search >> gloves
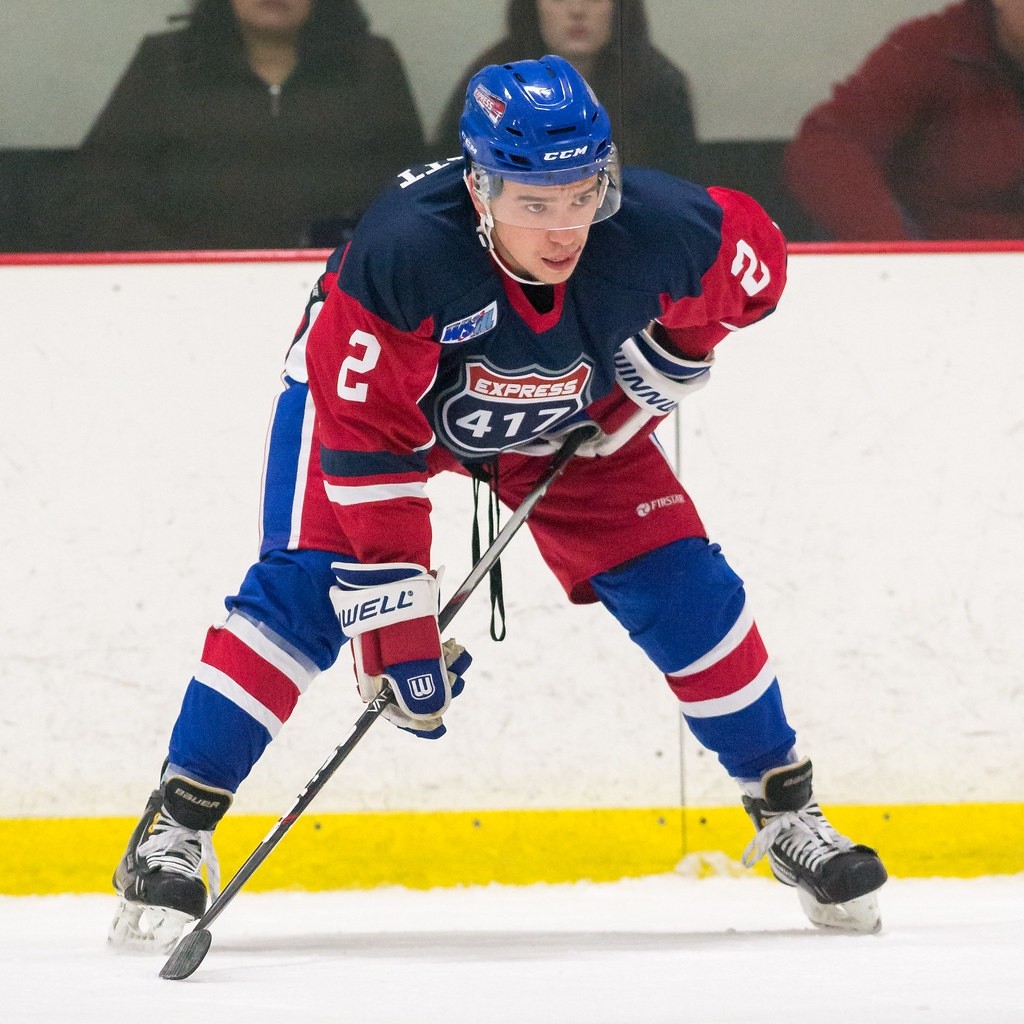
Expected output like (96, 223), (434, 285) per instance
(329, 560), (472, 740)
(540, 329), (715, 459)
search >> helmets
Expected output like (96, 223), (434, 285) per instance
(458, 54), (612, 205)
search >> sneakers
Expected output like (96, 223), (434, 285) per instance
(740, 755), (888, 934)
(107, 753), (233, 958)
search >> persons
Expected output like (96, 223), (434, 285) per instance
(82, 0), (424, 257)
(114, 57), (890, 962)
(431, 1), (702, 179)
(777, 1), (1024, 240)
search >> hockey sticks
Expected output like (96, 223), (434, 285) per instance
(160, 426), (595, 978)
